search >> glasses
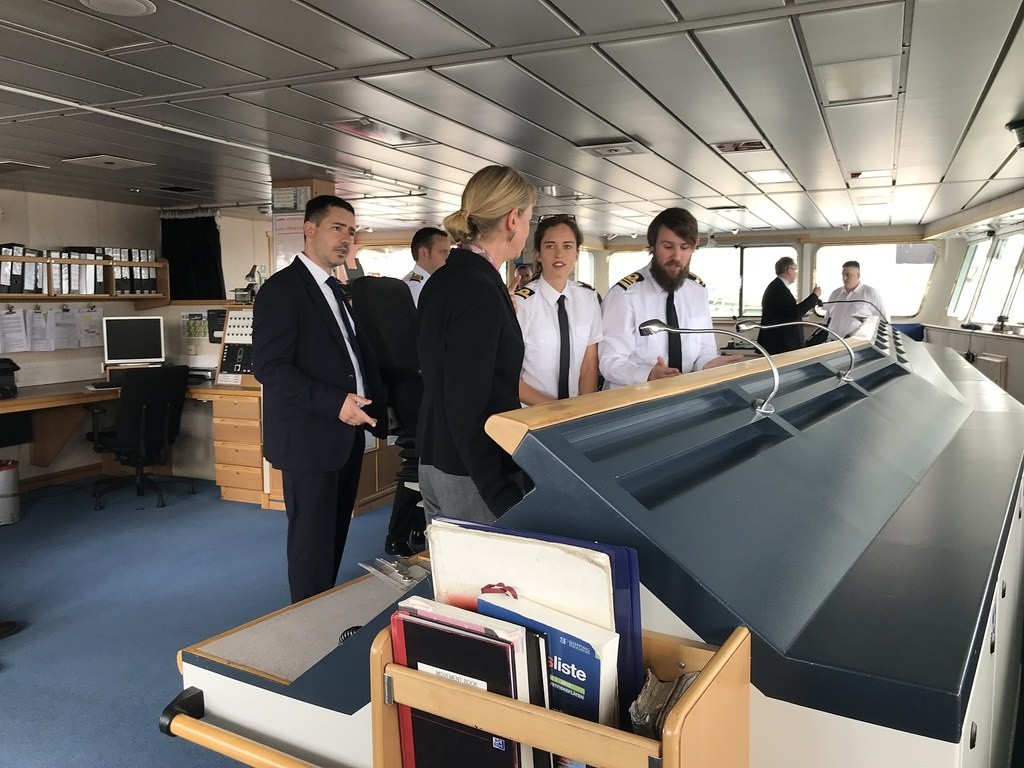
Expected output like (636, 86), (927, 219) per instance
(537, 213), (577, 225)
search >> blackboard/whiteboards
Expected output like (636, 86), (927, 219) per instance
(219, 342), (254, 374)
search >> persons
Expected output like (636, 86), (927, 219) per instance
(253, 195), (378, 604)
(414, 165), (537, 549)
(514, 265), (534, 288)
(385, 225), (451, 559)
(755, 257), (822, 355)
(514, 214), (604, 408)
(821, 260), (886, 341)
(597, 207), (744, 391)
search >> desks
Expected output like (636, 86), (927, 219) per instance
(719, 341), (763, 358)
(0, 378), (263, 505)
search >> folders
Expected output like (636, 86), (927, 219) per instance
(0, 242), (157, 294)
(430, 513), (644, 734)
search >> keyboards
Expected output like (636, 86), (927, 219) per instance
(91, 380), (121, 390)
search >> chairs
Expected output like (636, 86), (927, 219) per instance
(80, 365), (195, 511)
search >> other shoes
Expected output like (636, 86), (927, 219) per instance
(384, 535), (422, 557)
(0, 620), (23, 639)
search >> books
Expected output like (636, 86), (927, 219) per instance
(388, 591), (620, 768)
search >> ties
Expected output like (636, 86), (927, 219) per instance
(558, 295), (570, 400)
(666, 292), (682, 375)
(326, 276), (374, 411)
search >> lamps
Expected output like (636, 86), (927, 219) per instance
(245, 265), (261, 286)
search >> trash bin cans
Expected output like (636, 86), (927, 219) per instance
(0, 459), (21, 525)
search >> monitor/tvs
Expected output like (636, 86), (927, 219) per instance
(102, 316), (165, 367)
